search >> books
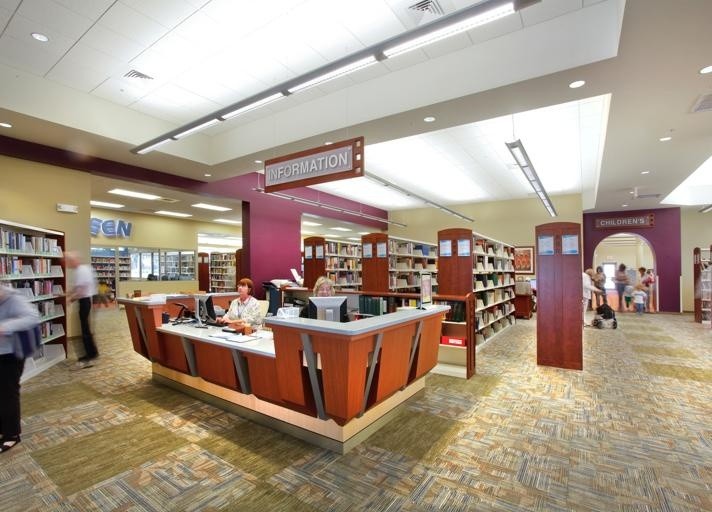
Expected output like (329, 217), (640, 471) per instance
(210, 254), (236, 292)
(358, 296), (466, 321)
(324, 242), (362, 290)
(0, 222), (131, 367)
(389, 240), (439, 307)
(473, 237), (516, 343)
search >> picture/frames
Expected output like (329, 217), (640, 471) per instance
(515, 246), (534, 274)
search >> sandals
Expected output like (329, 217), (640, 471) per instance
(1, 435), (20, 452)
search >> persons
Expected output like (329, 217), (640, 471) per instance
(0, 286), (41, 451)
(612, 263), (656, 314)
(216, 278), (261, 326)
(299, 276), (335, 318)
(583, 267), (603, 327)
(592, 267), (607, 308)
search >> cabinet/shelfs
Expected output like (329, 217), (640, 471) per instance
(91, 248), (243, 309)
(0, 219), (65, 385)
(535, 222), (584, 371)
(694, 245), (712, 323)
(281, 228), (515, 380)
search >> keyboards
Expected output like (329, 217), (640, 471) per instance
(208, 323), (225, 327)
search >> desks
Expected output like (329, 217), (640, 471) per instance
(515, 296), (533, 320)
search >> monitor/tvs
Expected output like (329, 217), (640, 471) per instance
(308, 296), (347, 322)
(194, 295), (217, 328)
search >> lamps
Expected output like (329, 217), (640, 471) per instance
(251, 173), (408, 228)
(129, 0), (544, 155)
(505, 139), (557, 218)
(365, 168), (475, 224)
